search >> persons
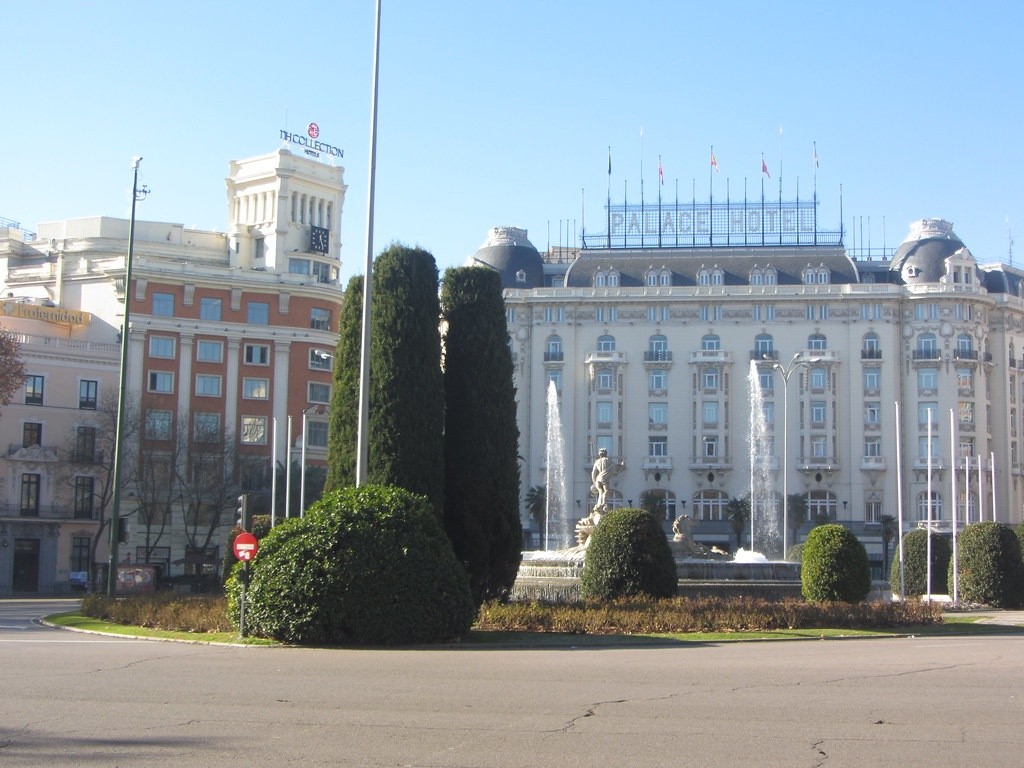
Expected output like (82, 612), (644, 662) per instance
(590, 447), (626, 514)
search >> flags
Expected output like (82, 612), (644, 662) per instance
(814, 148), (819, 167)
(762, 159), (771, 179)
(711, 152), (720, 172)
(658, 161), (664, 185)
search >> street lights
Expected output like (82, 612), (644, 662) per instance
(762, 351), (821, 561)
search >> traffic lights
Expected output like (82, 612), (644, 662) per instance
(236, 494), (253, 532)
